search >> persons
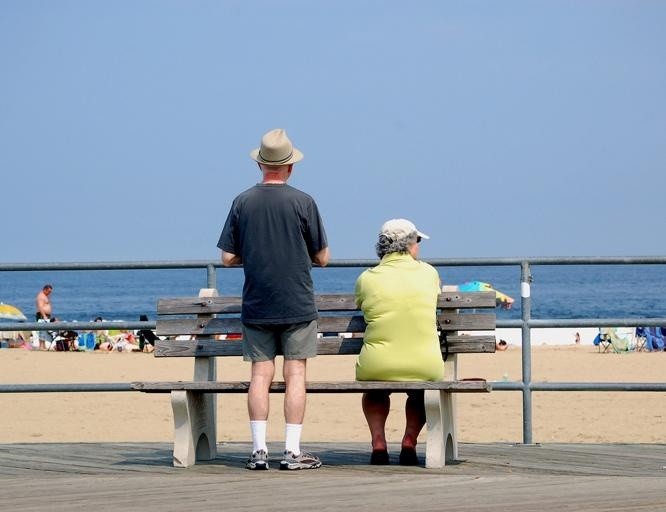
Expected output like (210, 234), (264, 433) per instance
(353, 218), (447, 466)
(214, 129), (330, 471)
(575, 332), (581, 344)
(34, 284), (54, 351)
(11, 316), (154, 353)
(495, 339), (509, 351)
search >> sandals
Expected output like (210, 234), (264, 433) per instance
(371, 450), (390, 464)
(398, 447), (419, 464)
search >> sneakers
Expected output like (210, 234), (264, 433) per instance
(279, 449), (323, 469)
(245, 449), (269, 470)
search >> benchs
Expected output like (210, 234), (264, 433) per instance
(130, 290), (497, 468)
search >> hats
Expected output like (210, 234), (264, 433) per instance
(378, 218), (431, 244)
(249, 128), (304, 166)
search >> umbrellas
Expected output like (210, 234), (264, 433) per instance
(0, 301), (28, 322)
(442, 280), (515, 314)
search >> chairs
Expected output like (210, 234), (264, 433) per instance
(48, 330), (78, 352)
(636, 326), (666, 352)
(599, 326), (635, 353)
